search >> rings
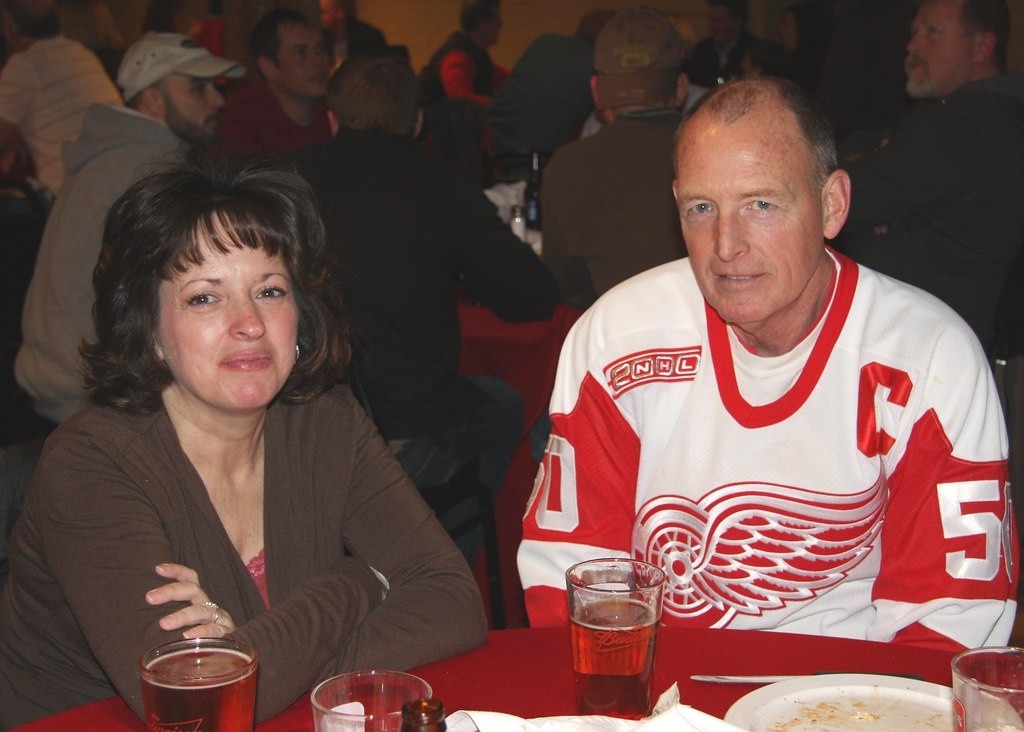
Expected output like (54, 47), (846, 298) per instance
(205, 602), (218, 622)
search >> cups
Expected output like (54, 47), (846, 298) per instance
(310, 669), (433, 732)
(141, 637), (258, 732)
(565, 557), (666, 721)
(951, 647), (1024, 732)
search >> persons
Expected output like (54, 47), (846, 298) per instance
(543, 8), (694, 306)
(516, 76), (1018, 652)
(0, 0), (1023, 569)
(281, 52), (556, 563)
(0, 165), (487, 732)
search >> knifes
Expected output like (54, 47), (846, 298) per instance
(690, 670), (925, 683)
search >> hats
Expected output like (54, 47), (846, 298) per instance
(116, 33), (244, 103)
(594, 5), (681, 109)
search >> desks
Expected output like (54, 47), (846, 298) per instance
(11, 623), (1024, 732)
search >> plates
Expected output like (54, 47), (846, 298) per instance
(723, 673), (952, 732)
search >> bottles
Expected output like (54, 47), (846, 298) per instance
(401, 698), (447, 732)
